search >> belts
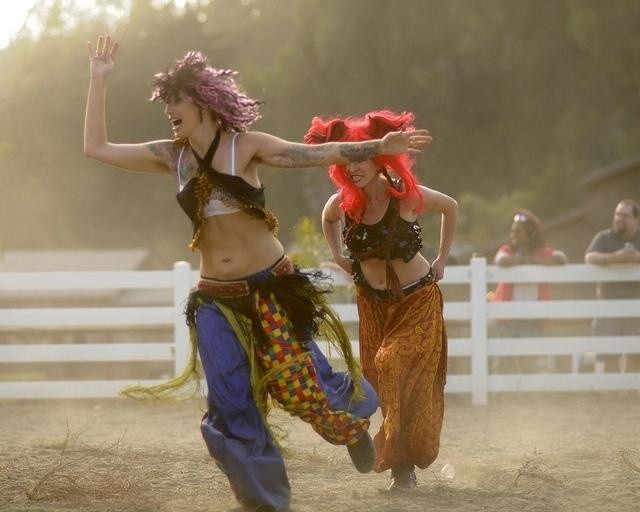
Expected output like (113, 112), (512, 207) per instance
(349, 267), (433, 299)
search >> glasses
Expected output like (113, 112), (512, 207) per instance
(512, 214), (530, 225)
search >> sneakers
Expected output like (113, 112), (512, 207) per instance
(347, 432), (377, 474)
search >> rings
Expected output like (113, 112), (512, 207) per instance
(95, 51), (102, 54)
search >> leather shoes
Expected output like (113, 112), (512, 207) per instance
(389, 465), (416, 492)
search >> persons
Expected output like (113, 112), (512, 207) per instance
(492, 208), (568, 374)
(583, 198), (640, 397)
(305, 110), (459, 495)
(81, 34), (433, 510)
